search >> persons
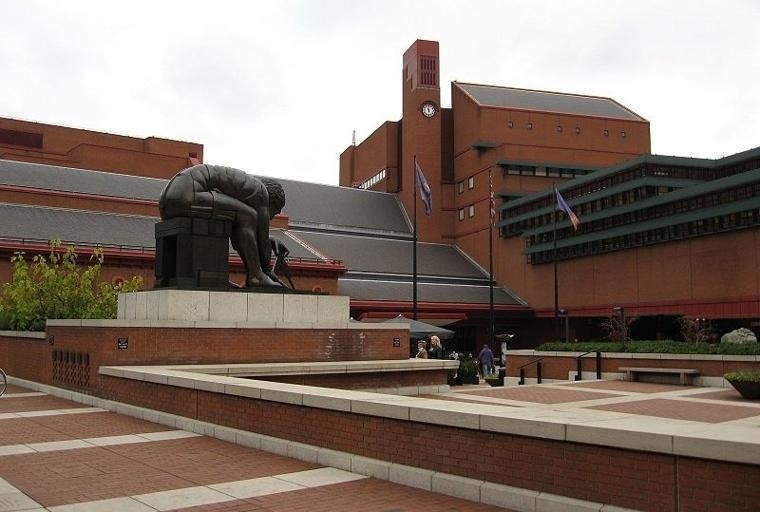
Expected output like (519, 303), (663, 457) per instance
(478, 343), (494, 378)
(456, 352), (466, 361)
(415, 340), (428, 359)
(157, 165), (291, 290)
(427, 335), (443, 359)
(450, 350), (460, 360)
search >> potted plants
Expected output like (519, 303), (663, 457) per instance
(724, 370), (760, 399)
(484, 372), (499, 387)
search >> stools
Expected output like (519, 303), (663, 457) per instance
(154, 216), (232, 290)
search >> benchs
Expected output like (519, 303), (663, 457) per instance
(618, 367), (700, 385)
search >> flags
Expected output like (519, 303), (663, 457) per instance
(413, 160), (434, 217)
(554, 186), (581, 232)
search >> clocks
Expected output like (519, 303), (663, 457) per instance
(422, 103), (435, 118)
